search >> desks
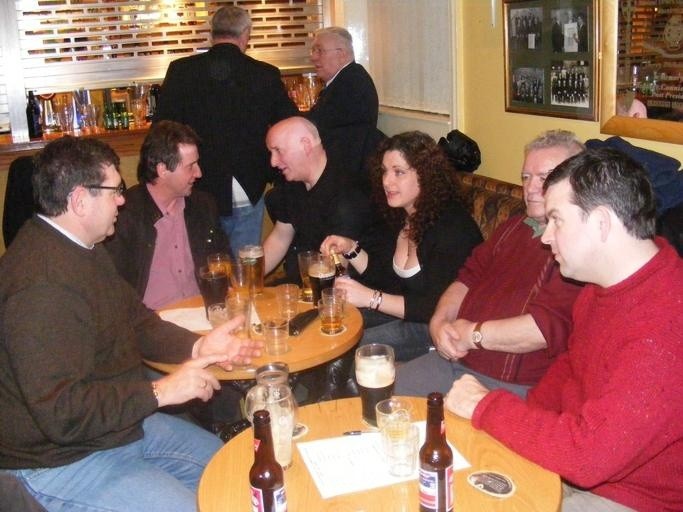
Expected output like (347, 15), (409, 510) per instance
(137, 284), (364, 405)
(196, 394), (564, 512)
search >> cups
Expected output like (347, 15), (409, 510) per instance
(352, 345), (398, 425)
(207, 252), (232, 278)
(207, 304), (230, 328)
(253, 359), (289, 398)
(297, 251), (315, 301)
(379, 424), (418, 476)
(131, 99), (145, 128)
(236, 245), (267, 295)
(199, 264), (225, 307)
(225, 290), (252, 328)
(322, 288), (345, 312)
(315, 300), (344, 336)
(245, 381), (293, 474)
(309, 256), (336, 304)
(261, 317), (292, 357)
(275, 283), (300, 318)
(373, 398), (413, 435)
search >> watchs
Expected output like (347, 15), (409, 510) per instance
(473, 320), (485, 352)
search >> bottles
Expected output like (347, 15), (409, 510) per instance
(630, 65), (639, 92)
(249, 408), (285, 512)
(25, 90), (42, 139)
(102, 100), (129, 130)
(641, 75), (653, 95)
(415, 391), (454, 512)
(58, 92), (75, 134)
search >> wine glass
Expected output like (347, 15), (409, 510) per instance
(90, 104), (99, 130)
(78, 101), (89, 129)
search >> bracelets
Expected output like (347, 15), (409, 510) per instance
(342, 241), (362, 261)
(150, 379), (161, 405)
(375, 289), (382, 312)
(367, 288), (379, 311)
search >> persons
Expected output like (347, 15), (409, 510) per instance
(259, 116), (393, 296)
(304, 131), (485, 401)
(616, 95), (649, 121)
(104, 121), (258, 440)
(1, 136), (264, 512)
(152, 7), (301, 282)
(367, 127), (590, 412)
(512, 12), (590, 108)
(442, 146), (683, 512)
(270, 27), (379, 190)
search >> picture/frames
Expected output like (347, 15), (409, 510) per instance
(502, 1), (596, 122)
(598, 0), (682, 145)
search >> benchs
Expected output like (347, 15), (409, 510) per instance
(394, 167), (525, 371)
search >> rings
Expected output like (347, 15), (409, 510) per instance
(202, 379), (208, 389)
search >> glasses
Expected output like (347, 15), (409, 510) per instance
(68, 184), (124, 197)
(311, 48), (342, 56)
